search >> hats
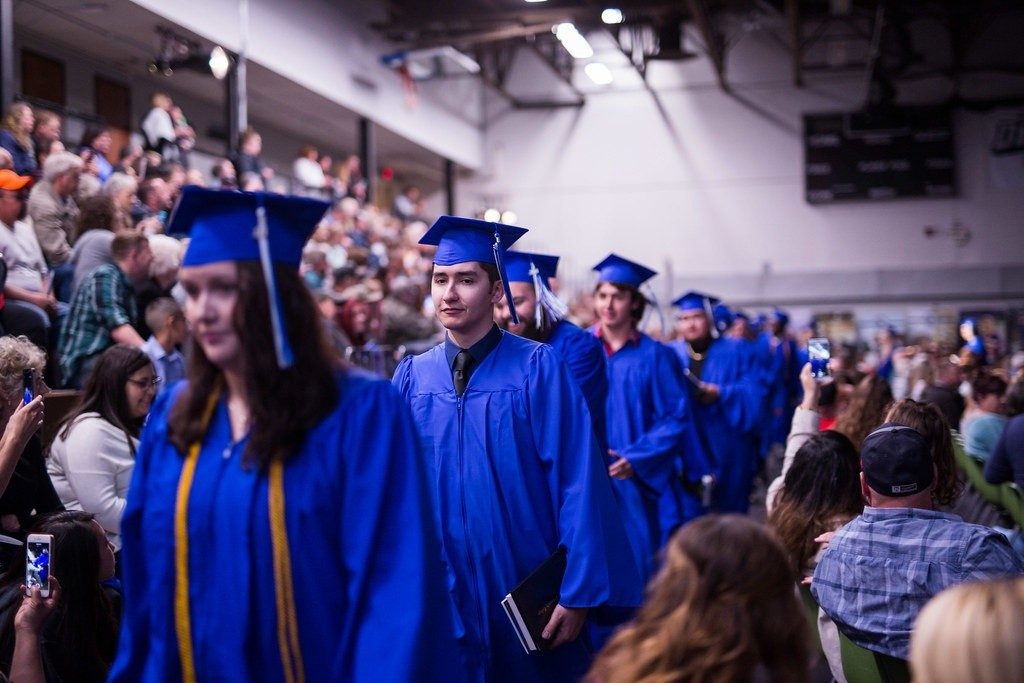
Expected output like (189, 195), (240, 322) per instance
(592, 254), (666, 338)
(503, 249), (568, 328)
(672, 292), (788, 339)
(0, 168), (33, 190)
(860, 422), (934, 498)
(164, 183), (333, 368)
(417, 214), (529, 324)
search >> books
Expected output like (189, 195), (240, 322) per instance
(501, 550), (567, 654)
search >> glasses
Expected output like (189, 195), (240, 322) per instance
(128, 374), (162, 388)
(2, 191), (30, 202)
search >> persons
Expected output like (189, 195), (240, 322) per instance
(0, 298), (188, 683)
(107, 183), (458, 683)
(391, 216), (645, 683)
(594, 253), (694, 654)
(34, 547), (48, 586)
(729, 308), (820, 484)
(495, 251), (609, 466)
(670, 292), (763, 515)
(582, 331), (1024, 683)
(0, 94), (597, 389)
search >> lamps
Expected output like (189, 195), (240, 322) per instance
(207, 45), (236, 82)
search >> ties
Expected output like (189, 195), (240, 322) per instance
(453, 353), (473, 398)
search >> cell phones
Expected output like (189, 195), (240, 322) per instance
(808, 337), (832, 383)
(23, 368), (37, 406)
(26, 533), (55, 598)
